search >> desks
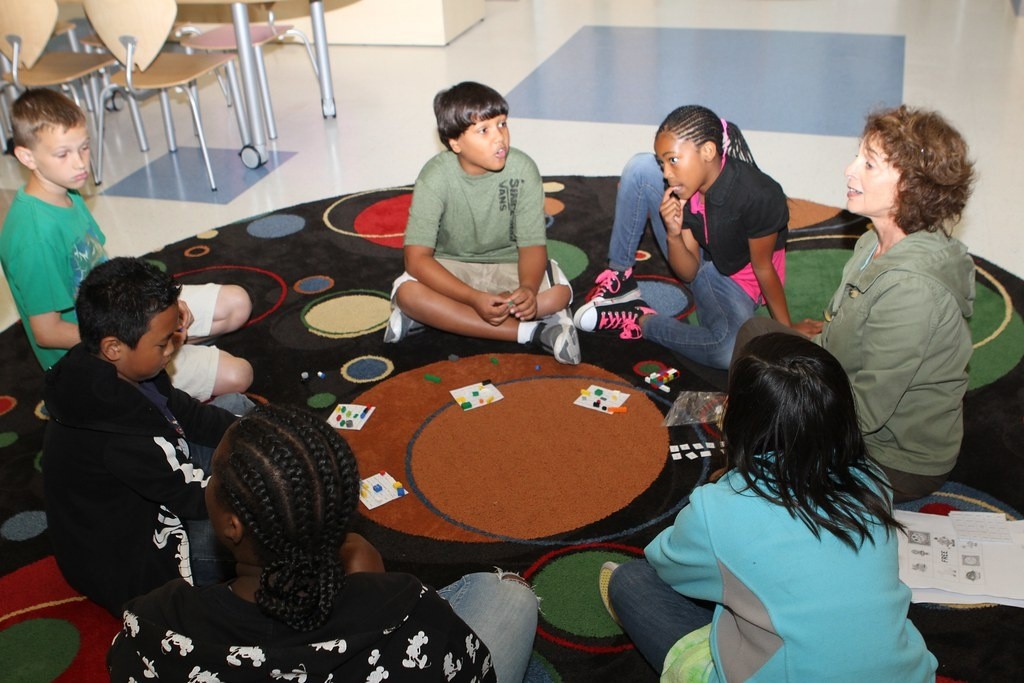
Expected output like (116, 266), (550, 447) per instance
(63, 0), (339, 168)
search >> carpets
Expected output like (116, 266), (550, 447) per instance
(0, 175), (1024, 683)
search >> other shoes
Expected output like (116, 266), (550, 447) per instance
(599, 562), (622, 627)
(574, 299), (657, 340)
(590, 267), (641, 302)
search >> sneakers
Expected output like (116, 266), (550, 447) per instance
(531, 308), (580, 367)
(384, 307), (426, 344)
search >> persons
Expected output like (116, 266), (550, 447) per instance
(597, 332), (940, 682)
(728, 104), (977, 503)
(35, 255), (260, 612)
(389, 82), (585, 365)
(1, 87), (254, 401)
(570, 104), (818, 373)
(101, 401), (545, 683)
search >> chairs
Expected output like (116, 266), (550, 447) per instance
(0, 0), (319, 191)
